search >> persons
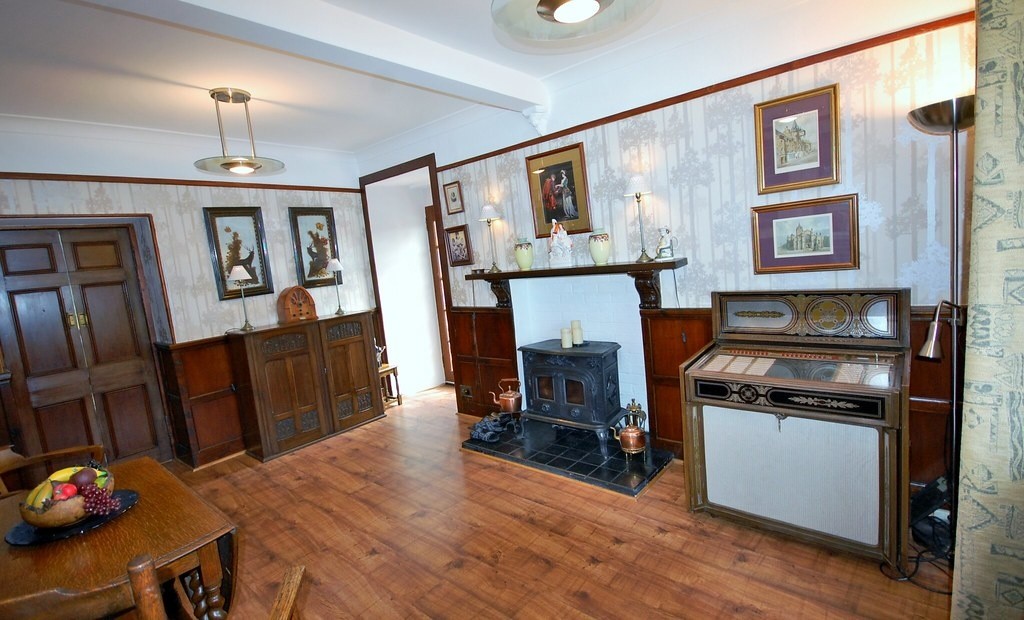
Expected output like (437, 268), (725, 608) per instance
(374, 337), (385, 367)
(546, 218), (573, 260)
(656, 224), (673, 257)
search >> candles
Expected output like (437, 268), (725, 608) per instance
(561, 319), (582, 349)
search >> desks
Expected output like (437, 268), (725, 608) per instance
(0, 457), (239, 620)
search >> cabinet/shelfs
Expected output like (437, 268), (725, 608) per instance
(225, 308), (388, 463)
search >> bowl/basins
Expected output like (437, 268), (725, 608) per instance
(20, 468), (115, 527)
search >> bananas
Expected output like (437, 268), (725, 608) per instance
(26, 467), (108, 509)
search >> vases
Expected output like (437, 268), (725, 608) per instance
(514, 237), (533, 271)
(589, 228), (610, 265)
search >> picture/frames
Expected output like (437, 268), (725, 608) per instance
(750, 193), (860, 275)
(525, 141), (594, 239)
(444, 224), (475, 267)
(443, 181), (465, 216)
(288, 207), (343, 289)
(202, 206), (274, 301)
(754, 82), (840, 195)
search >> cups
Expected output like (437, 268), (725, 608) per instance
(561, 328), (572, 348)
(571, 321), (583, 345)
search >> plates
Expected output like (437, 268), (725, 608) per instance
(4, 490), (140, 545)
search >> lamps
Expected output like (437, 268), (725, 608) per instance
(478, 206), (502, 273)
(906, 95), (975, 548)
(228, 265), (256, 331)
(491, 0), (660, 55)
(326, 259), (346, 315)
(192, 87), (286, 177)
(623, 175), (654, 263)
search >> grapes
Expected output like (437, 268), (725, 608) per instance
(79, 483), (121, 514)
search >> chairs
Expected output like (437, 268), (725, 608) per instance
(379, 363), (402, 412)
(0, 443), (106, 500)
(0, 553), (168, 619)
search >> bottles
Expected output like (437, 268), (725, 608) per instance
(589, 226), (609, 266)
(514, 237), (533, 271)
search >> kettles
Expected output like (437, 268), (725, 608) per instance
(610, 411), (647, 454)
(489, 378), (522, 412)
